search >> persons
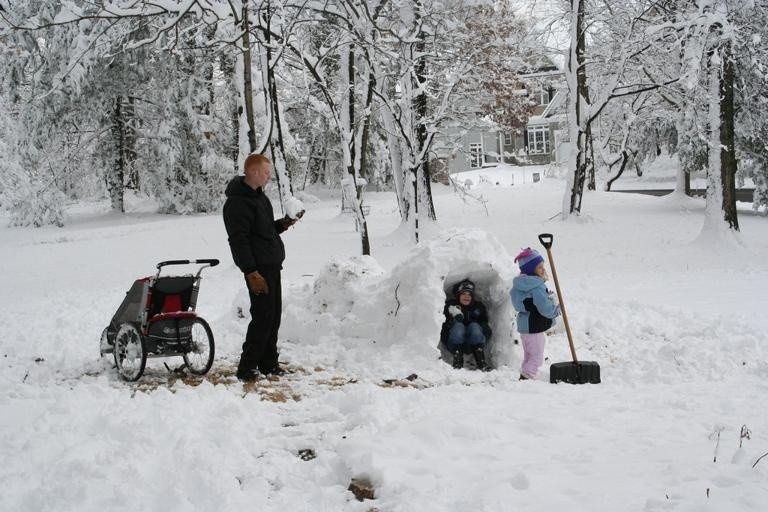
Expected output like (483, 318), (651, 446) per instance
(222, 154), (306, 382)
(508, 248), (560, 383)
(441, 280), (493, 372)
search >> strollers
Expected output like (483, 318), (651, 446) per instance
(100, 258), (219, 382)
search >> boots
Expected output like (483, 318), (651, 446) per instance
(452, 344), (463, 368)
(470, 343), (491, 371)
(237, 354), (286, 382)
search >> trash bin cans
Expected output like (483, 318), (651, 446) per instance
(533, 173), (540, 182)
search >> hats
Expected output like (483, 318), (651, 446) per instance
(455, 280), (474, 295)
(514, 247), (544, 275)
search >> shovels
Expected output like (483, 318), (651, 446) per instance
(539, 233), (600, 384)
